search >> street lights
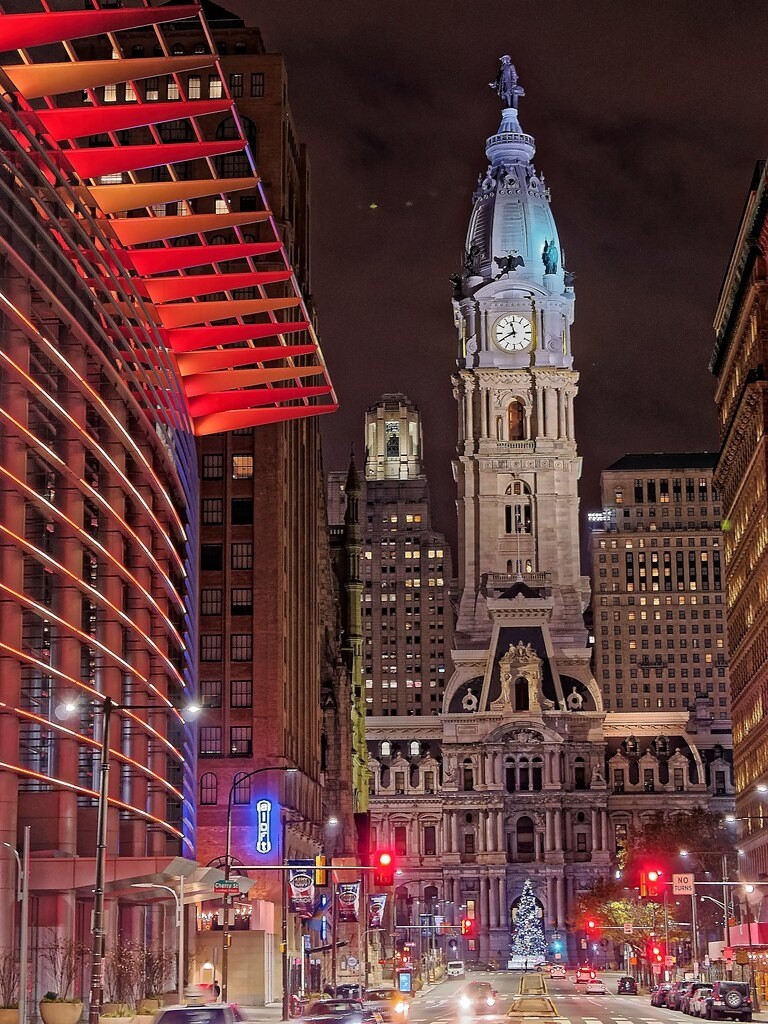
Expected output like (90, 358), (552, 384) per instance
(393, 877), (431, 988)
(280, 814), (338, 1021)
(130, 874), (185, 1006)
(222, 766), (298, 1004)
(357, 925), (386, 999)
(700, 885), (735, 919)
(677, 849), (744, 981)
(64, 697), (204, 1024)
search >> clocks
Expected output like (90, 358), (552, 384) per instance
(490, 311), (533, 354)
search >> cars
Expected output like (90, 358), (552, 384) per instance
(464, 960), (496, 972)
(697, 994), (714, 1020)
(650, 982), (674, 1008)
(534, 961), (558, 972)
(361, 986), (409, 1024)
(336, 984), (366, 1000)
(585, 979), (606, 996)
(463, 981), (499, 1015)
(688, 988), (714, 1017)
(293, 998), (385, 1024)
(550, 965), (566, 979)
(576, 967), (594, 984)
(156, 1004), (244, 1024)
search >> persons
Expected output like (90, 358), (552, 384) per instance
(211, 981), (220, 1000)
(488, 54), (524, 108)
(323, 985), (334, 998)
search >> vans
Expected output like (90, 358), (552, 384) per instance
(447, 961), (466, 980)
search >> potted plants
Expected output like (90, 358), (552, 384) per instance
(411, 978), (424, 992)
(0, 926), (179, 1024)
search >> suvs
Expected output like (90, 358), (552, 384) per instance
(617, 976), (638, 995)
(707, 981), (753, 1023)
(665, 980), (699, 1011)
(679, 982), (714, 1015)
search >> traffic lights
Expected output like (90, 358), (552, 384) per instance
(464, 919), (473, 936)
(647, 864), (660, 898)
(586, 918), (596, 934)
(649, 943), (665, 962)
(374, 849), (396, 887)
